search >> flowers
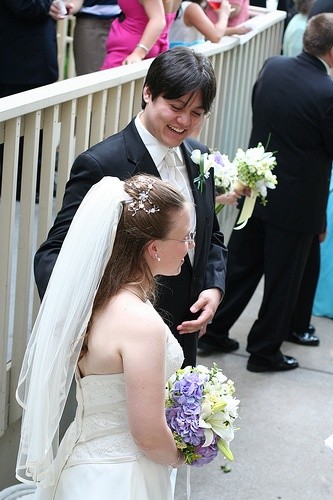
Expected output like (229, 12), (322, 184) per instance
(191, 149), (214, 193)
(209, 134), (278, 231)
(165, 361), (240, 474)
